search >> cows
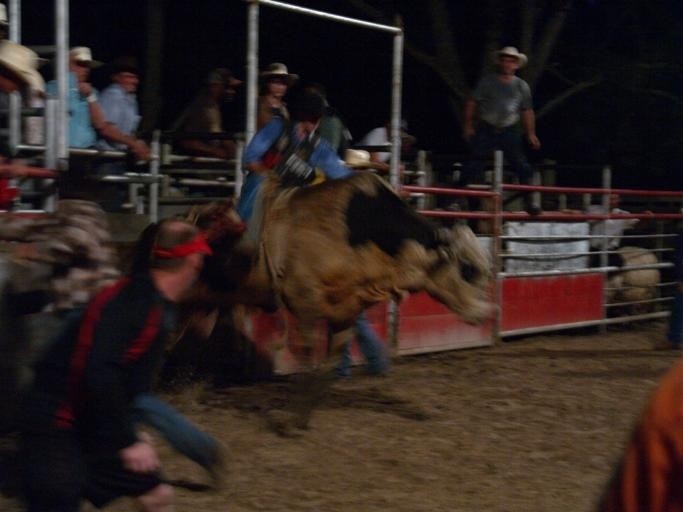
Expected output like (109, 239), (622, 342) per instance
(171, 168), (499, 441)
(577, 245), (660, 334)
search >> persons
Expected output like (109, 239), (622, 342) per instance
(337, 305), (384, 383)
(350, 115), (416, 183)
(594, 360), (682, 512)
(665, 208), (683, 349)
(13, 215), (228, 511)
(2, 38), (151, 214)
(584, 190), (653, 279)
(458, 45), (541, 209)
(172, 60), (355, 252)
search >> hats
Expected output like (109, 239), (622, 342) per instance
(0, 39), (45, 92)
(263, 63), (298, 81)
(71, 46), (105, 68)
(490, 47), (528, 68)
